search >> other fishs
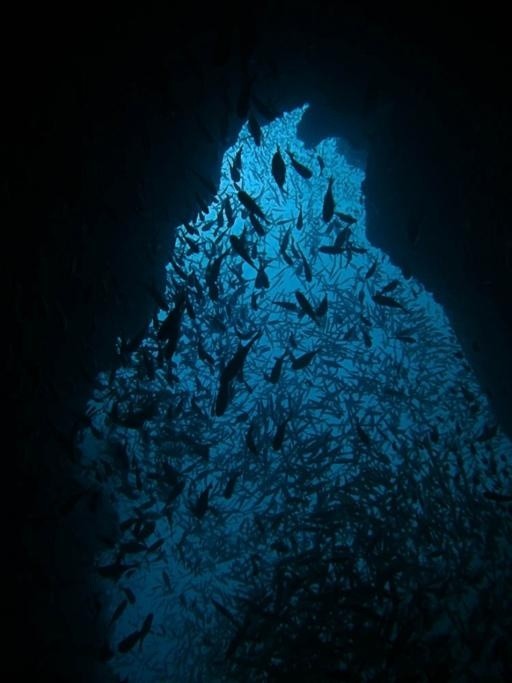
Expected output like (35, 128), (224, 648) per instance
(72, 106), (512, 683)
